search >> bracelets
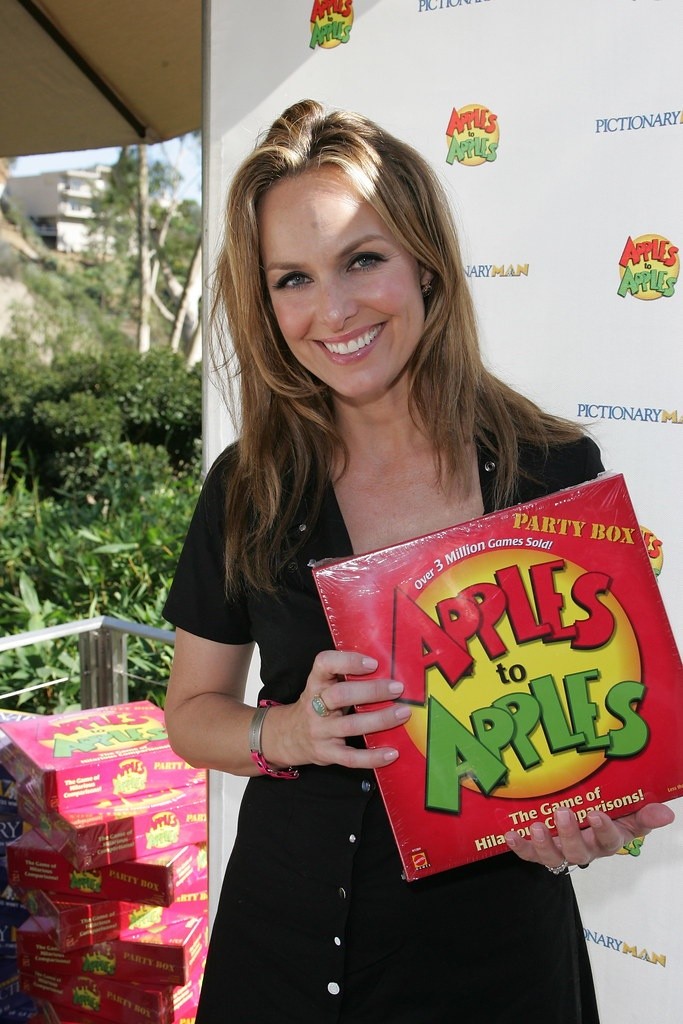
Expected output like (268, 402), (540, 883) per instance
(248, 700), (301, 780)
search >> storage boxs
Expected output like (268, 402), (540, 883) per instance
(16, 911), (209, 987)
(307, 470), (683, 882)
(9, 872), (208, 954)
(36, 995), (202, 1024)
(6, 829), (208, 908)
(17, 783), (210, 872)
(0, 700), (206, 813)
(18, 951), (208, 1024)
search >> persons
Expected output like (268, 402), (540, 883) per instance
(162, 100), (675, 1024)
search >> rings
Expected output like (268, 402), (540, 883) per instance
(313, 691), (337, 718)
(545, 859), (568, 876)
